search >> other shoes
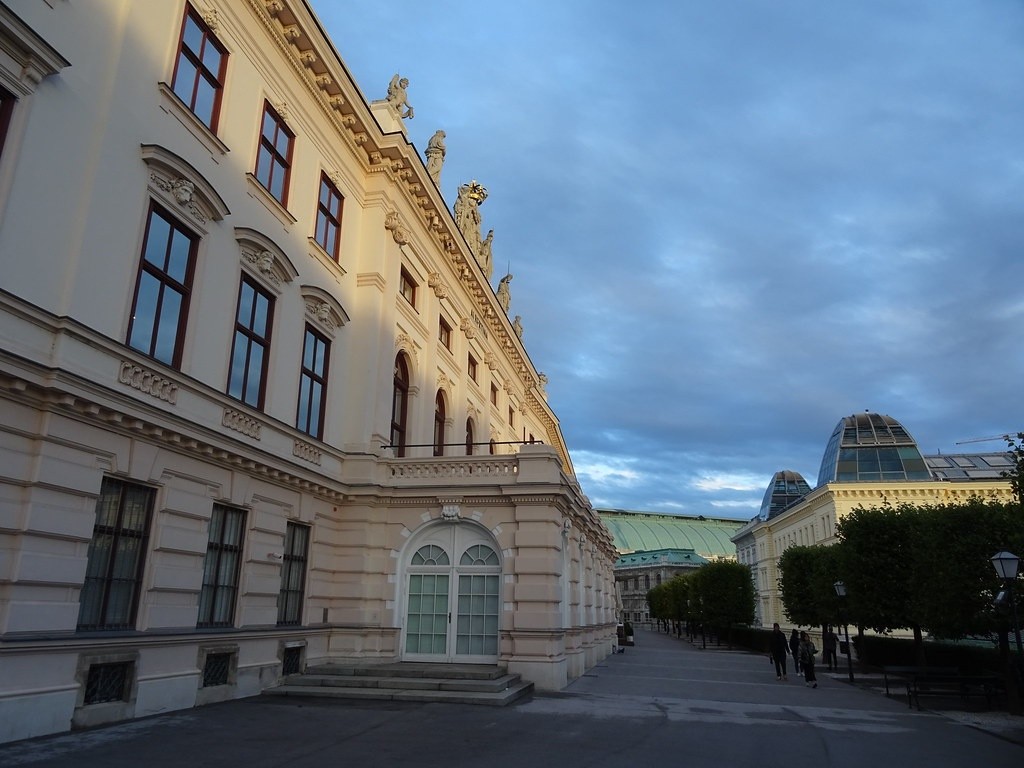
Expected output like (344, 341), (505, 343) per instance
(806, 682), (809, 687)
(777, 676), (781, 679)
(784, 674), (787, 679)
(812, 683), (817, 688)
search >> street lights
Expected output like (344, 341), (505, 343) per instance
(833, 579), (855, 681)
(989, 542), (1024, 665)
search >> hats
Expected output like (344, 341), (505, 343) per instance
(792, 629), (800, 634)
(829, 628), (833, 631)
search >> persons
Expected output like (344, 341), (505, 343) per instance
(424, 129), (447, 188)
(386, 74), (411, 117)
(767, 623), (790, 680)
(498, 274), (513, 311)
(826, 627), (840, 671)
(513, 316), (523, 337)
(797, 634), (818, 688)
(789, 629), (805, 674)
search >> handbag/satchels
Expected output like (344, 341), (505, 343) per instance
(770, 652), (773, 664)
